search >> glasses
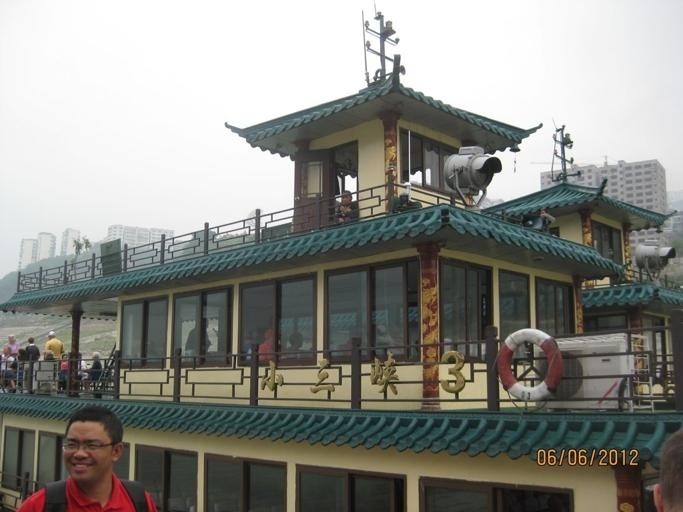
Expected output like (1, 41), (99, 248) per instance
(62, 438), (113, 451)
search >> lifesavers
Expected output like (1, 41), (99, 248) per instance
(496, 328), (563, 402)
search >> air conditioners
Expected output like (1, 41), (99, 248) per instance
(534, 331), (634, 412)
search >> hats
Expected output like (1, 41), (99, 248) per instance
(47, 331), (56, 337)
(265, 329), (281, 338)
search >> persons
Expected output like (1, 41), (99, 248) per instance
(652, 427), (682, 512)
(245, 327), (277, 361)
(0, 329), (102, 399)
(14, 404), (159, 511)
(335, 190), (359, 226)
(283, 330), (308, 360)
(184, 318), (213, 353)
